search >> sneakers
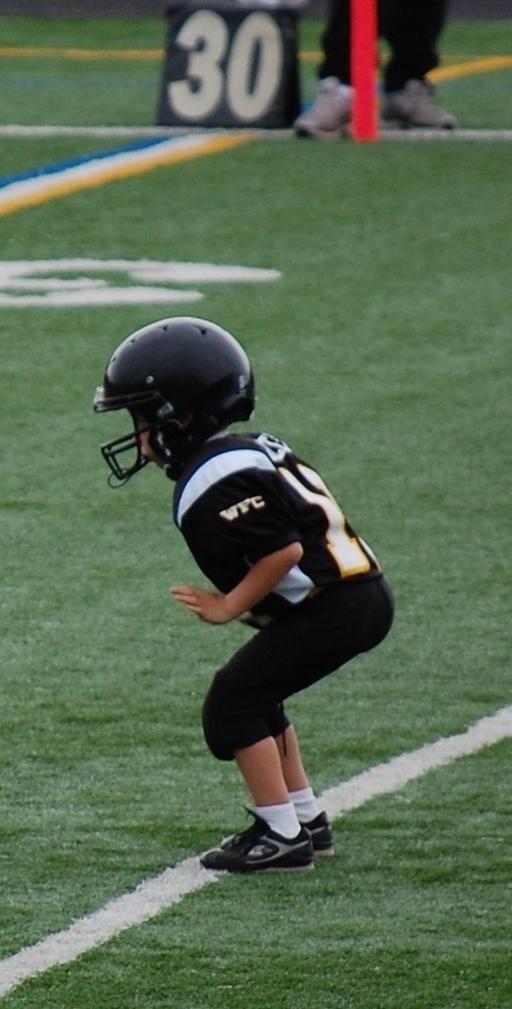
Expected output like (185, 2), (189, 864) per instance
(292, 74), (355, 142)
(196, 810), (336, 875)
(381, 80), (460, 133)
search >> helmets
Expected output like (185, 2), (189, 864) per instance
(90, 313), (256, 485)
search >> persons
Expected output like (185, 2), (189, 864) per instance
(294, 1), (459, 141)
(92, 315), (396, 873)
